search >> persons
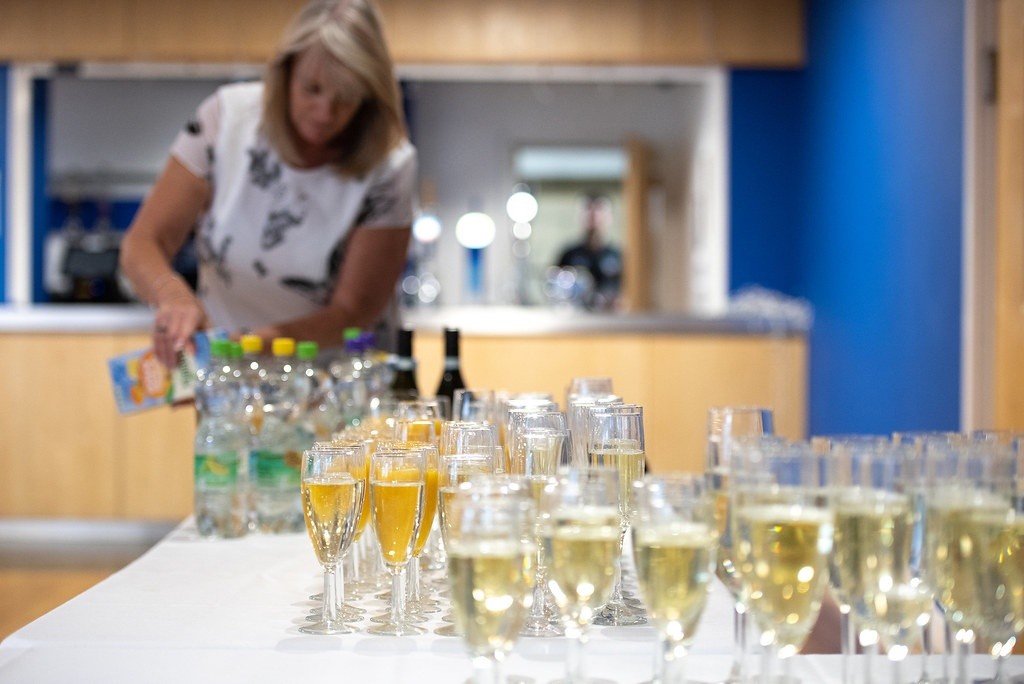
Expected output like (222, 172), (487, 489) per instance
(556, 194), (622, 311)
(121, 0), (422, 372)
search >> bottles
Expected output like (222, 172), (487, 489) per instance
(192, 325), (472, 538)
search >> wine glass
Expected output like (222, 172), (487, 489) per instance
(298, 375), (1024, 684)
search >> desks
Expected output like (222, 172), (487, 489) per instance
(0, 508), (1024, 684)
